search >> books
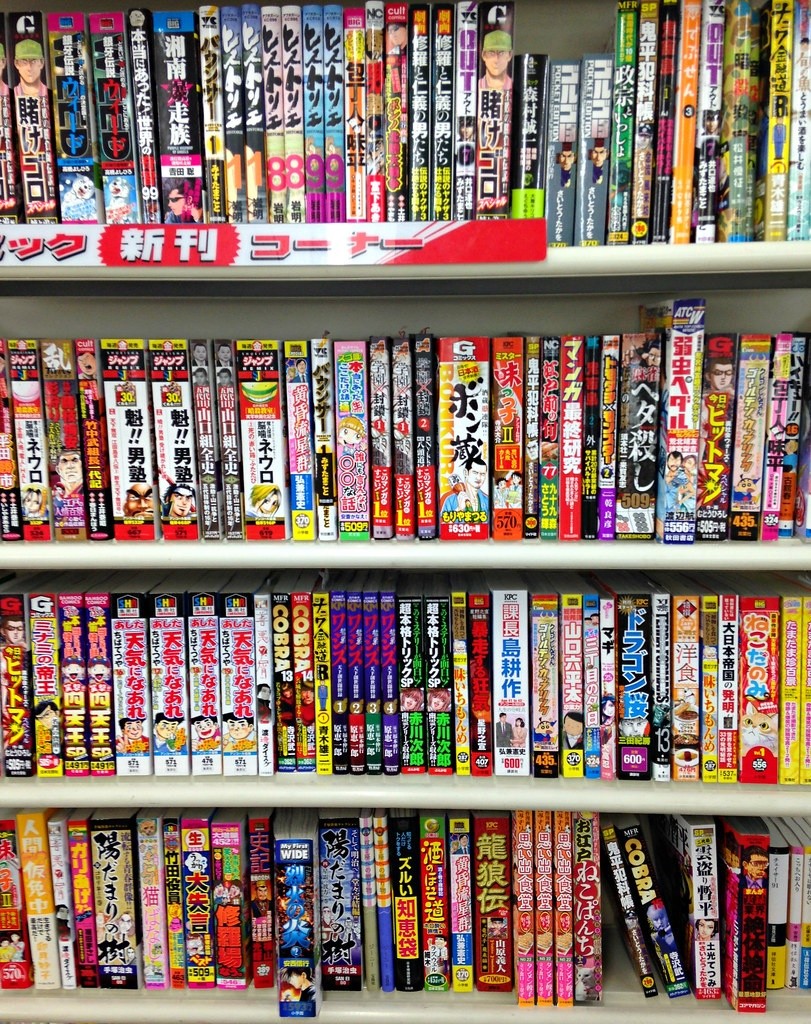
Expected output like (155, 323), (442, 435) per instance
(0, 571), (811, 789)
(0, 298), (811, 543)
(0, 808), (811, 1018)
(0, 0), (811, 249)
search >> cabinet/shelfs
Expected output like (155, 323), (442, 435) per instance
(0, 241), (811, 1024)
